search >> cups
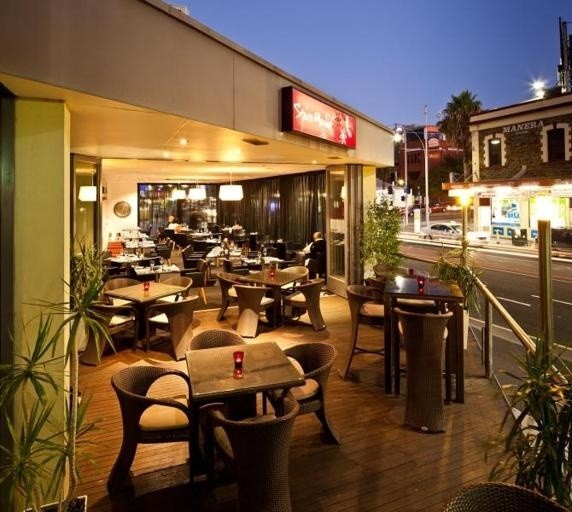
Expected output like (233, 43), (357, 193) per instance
(233, 351), (243, 368)
(418, 288), (424, 296)
(144, 281), (149, 291)
(234, 369), (243, 379)
(416, 276), (424, 288)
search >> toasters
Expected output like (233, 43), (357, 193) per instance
(150, 259), (172, 271)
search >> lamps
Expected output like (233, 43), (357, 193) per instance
(171, 161), (187, 201)
(187, 159), (208, 203)
(219, 154), (246, 202)
(79, 161), (98, 204)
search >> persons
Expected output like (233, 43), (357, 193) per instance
(304, 231), (324, 267)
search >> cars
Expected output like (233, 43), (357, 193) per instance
(138, 195), (217, 221)
(421, 222), (492, 250)
(387, 201), (462, 217)
(269, 193), (282, 213)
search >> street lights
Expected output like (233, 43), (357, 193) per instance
(393, 126), (409, 225)
(394, 105), (432, 228)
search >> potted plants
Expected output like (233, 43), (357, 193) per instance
(355, 191), (408, 280)
(39, 238), (115, 367)
(0, 254), (114, 512)
(433, 244), (478, 350)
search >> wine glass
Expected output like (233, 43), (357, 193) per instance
(258, 248), (267, 262)
(120, 248), (141, 259)
(221, 242), (225, 251)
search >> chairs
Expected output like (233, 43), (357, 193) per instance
(341, 264), (465, 433)
(80, 225), (339, 512)
